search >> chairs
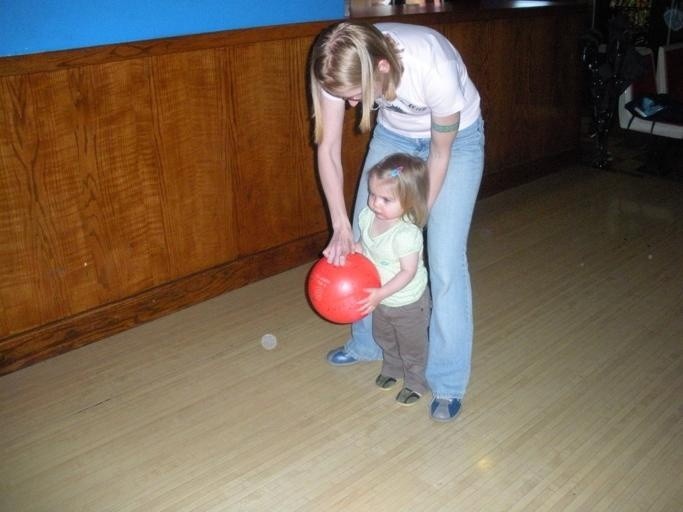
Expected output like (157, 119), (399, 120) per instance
(656, 42), (683, 103)
(616, 47), (683, 177)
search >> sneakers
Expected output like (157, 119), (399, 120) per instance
(375, 373), (398, 391)
(395, 386), (420, 405)
(326, 346), (358, 365)
(429, 397), (464, 422)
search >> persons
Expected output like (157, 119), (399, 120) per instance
(306, 18), (485, 422)
(345, 151), (432, 405)
